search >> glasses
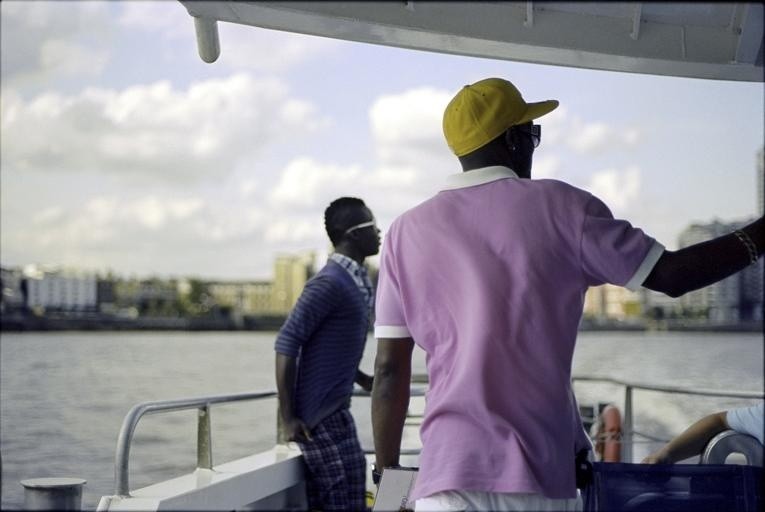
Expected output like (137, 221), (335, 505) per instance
(516, 123), (543, 148)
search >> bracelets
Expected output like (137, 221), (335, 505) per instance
(731, 229), (757, 266)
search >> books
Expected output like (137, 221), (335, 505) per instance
(373, 465), (419, 512)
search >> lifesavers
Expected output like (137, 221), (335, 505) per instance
(595, 406), (623, 461)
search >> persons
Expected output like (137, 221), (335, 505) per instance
(275, 199), (380, 512)
(636, 403), (765, 486)
(370, 79), (765, 512)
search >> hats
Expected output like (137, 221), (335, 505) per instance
(441, 78), (559, 157)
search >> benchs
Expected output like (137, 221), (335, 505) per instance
(590, 462), (765, 512)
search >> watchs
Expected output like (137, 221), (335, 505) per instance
(371, 462), (382, 485)
